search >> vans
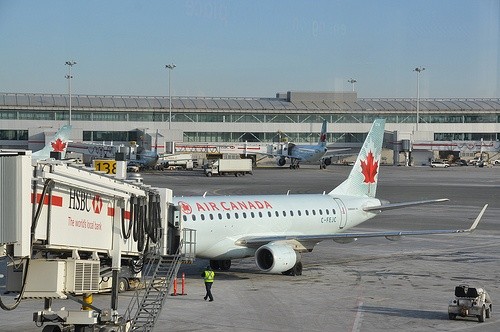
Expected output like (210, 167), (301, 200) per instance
(455, 159), (467, 165)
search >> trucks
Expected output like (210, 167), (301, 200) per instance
(204, 158), (253, 177)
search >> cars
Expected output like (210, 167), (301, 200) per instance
(446, 285), (493, 322)
(431, 161), (450, 168)
(127, 161), (139, 173)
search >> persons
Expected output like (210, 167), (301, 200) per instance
(201, 266), (216, 301)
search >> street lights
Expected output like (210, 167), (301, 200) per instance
(63, 60), (78, 126)
(163, 64), (176, 130)
(348, 79), (357, 93)
(410, 67), (426, 131)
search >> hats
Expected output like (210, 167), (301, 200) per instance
(207, 266), (211, 271)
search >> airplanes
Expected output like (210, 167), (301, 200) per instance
(130, 129), (198, 172)
(172, 118), (489, 277)
(243, 118), (352, 170)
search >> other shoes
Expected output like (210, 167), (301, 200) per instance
(204, 297), (206, 300)
(209, 299), (213, 301)
